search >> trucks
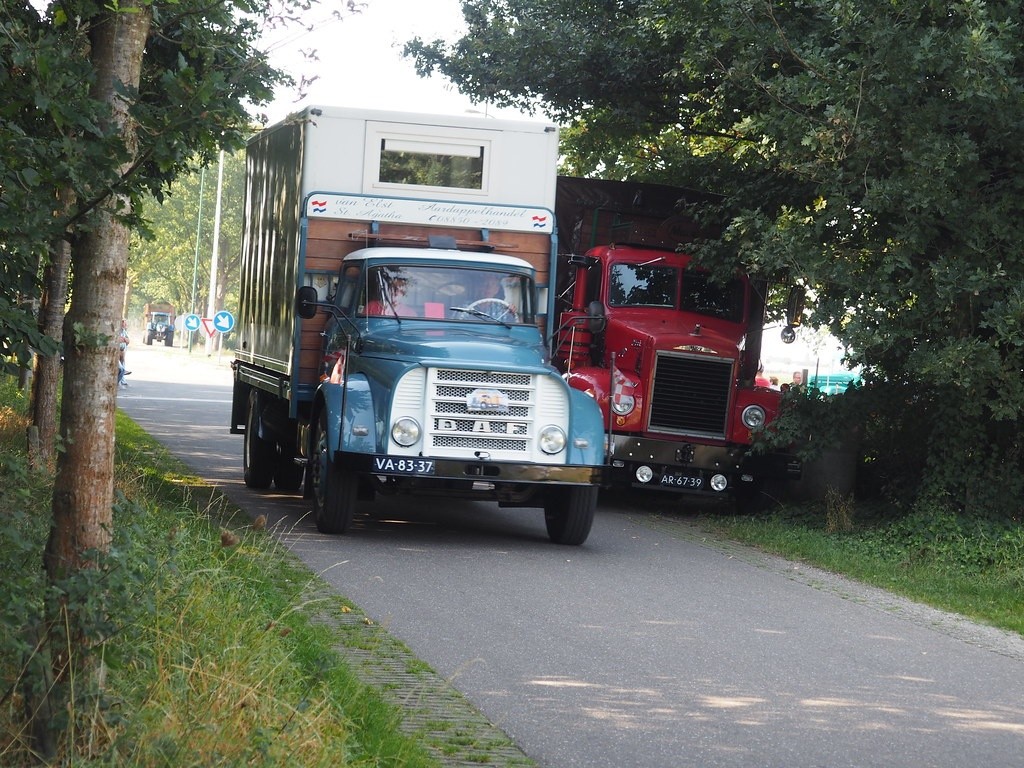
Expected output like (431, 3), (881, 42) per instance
(227, 104), (606, 545)
(142, 303), (175, 346)
(545, 174), (808, 514)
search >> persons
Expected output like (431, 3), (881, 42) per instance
(118, 318), (132, 386)
(365, 275), (418, 317)
(755, 364), (803, 393)
(453, 276), (519, 323)
